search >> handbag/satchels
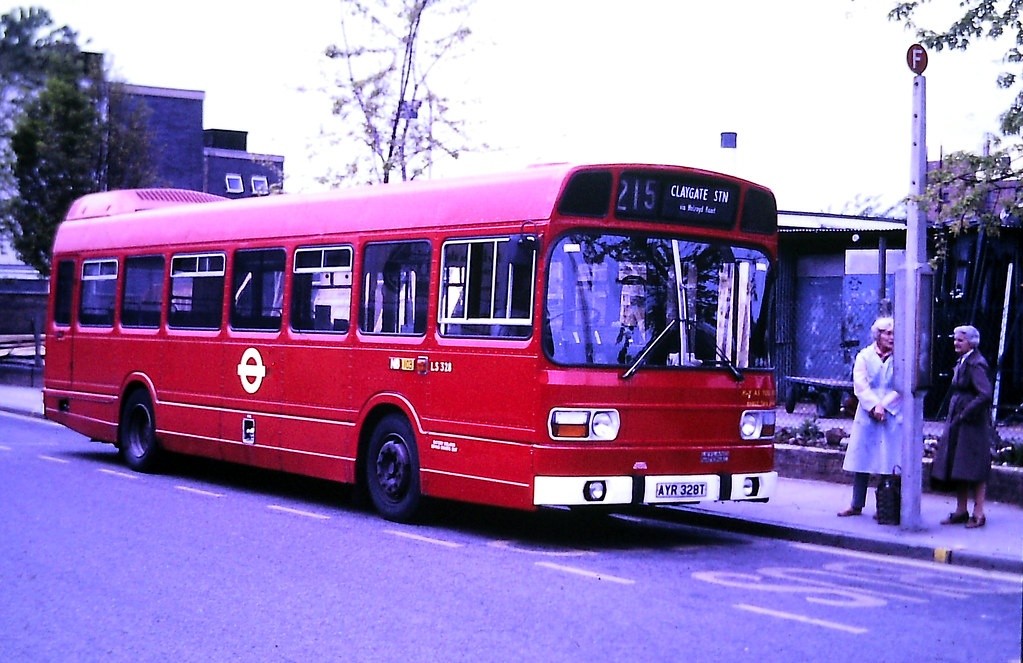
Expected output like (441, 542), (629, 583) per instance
(875, 466), (901, 526)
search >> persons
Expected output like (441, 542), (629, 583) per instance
(837, 317), (903, 519)
(931, 325), (994, 528)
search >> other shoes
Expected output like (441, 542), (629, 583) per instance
(965, 513), (986, 527)
(837, 506), (862, 516)
(940, 511), (969, 525)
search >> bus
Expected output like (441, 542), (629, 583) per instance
(41, 163), (780, 523)
